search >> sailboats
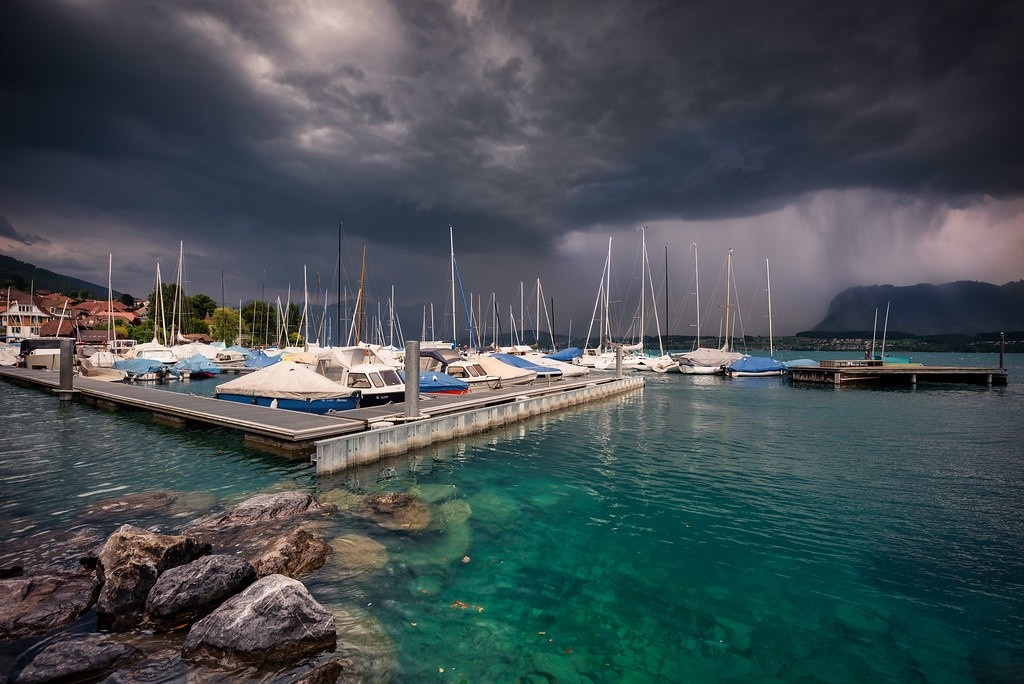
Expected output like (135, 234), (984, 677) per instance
(0, 222), (923, 412)
